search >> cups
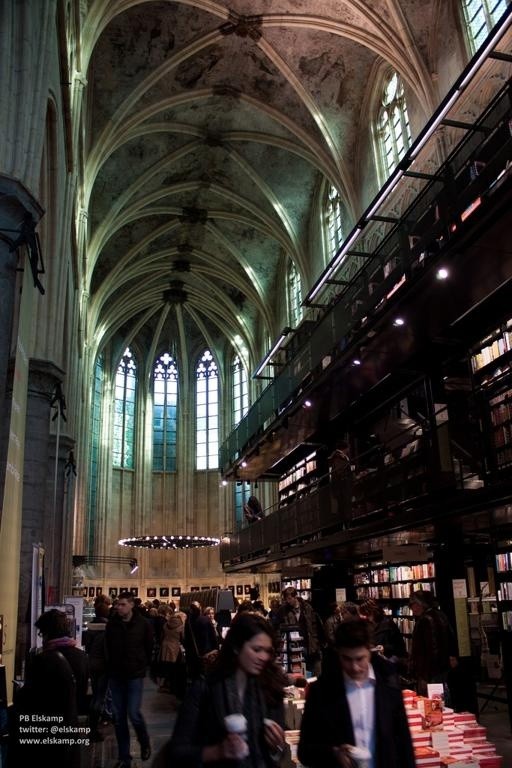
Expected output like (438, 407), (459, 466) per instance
(348, 747), (373, 768)
(225, 712), (251, 759)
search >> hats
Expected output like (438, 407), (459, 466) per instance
(32, 608), (68, 627)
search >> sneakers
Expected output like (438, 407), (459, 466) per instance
(115, 758), (132, 768)
(140, 742), (151, 761)
(86, 728), (105, 743)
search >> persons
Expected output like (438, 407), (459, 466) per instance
(328, 441), (353, 529)
(244, 496), (265, 524)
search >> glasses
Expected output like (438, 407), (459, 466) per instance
(36, 630), (45, 638)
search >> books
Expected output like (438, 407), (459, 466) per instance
(470, 331), (512, 466)
(278, 461), (317, 509)
